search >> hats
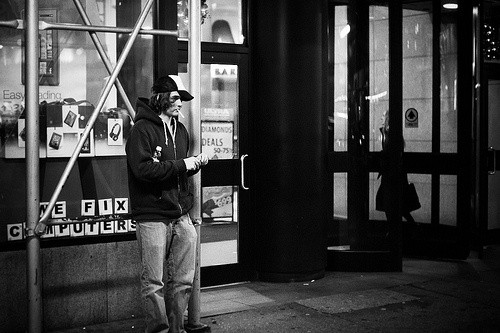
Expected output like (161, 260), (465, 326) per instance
(152, 74), (194, 102)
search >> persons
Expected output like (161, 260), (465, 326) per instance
(125, 74), (208, 333)
(376, 111), (421, 252)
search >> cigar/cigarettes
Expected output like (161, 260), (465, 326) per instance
(177, 109), (185, 118)
